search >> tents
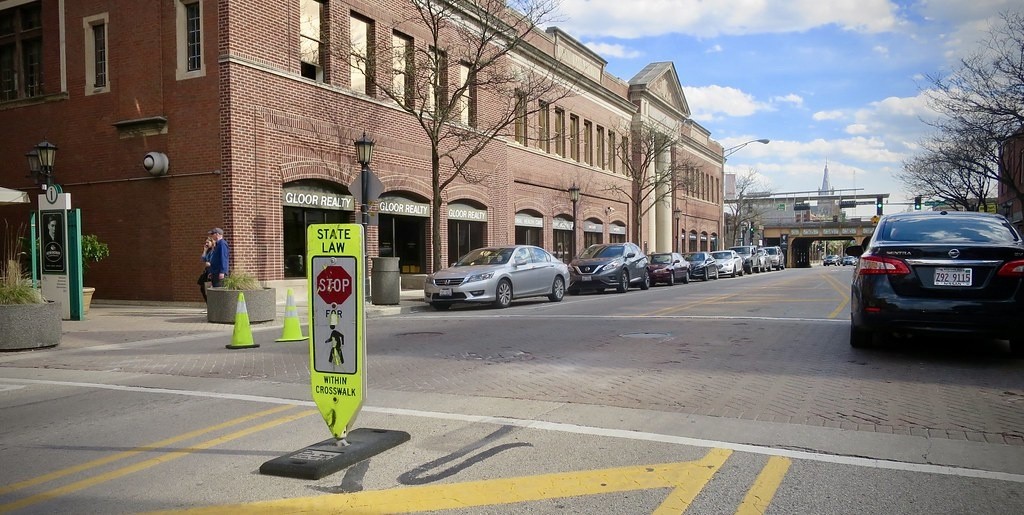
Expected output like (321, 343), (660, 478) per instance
(0, 187), (32, 205)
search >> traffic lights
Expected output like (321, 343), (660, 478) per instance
(915, 197), (921, 210)
(877, 196), (882, 215)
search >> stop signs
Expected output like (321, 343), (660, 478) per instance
(317, 265), (352, 304)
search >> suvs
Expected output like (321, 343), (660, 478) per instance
(567, 242), (650, 296)
(763, 246), (784, 271)
(729, 245), (759, 274)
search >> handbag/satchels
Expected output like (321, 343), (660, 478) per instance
(204, 277), (212, 295)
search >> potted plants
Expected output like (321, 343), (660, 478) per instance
(0, 259), (62, 351)
(207, 271), (276, 323)
(81, 234), (109, 314)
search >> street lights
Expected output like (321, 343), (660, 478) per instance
(674, 207), (682, 253)
(568, 181), (580, 258)
(352, 127), (377, 301)
(34, 137), (58, 187)
(722, 138), (770, 250)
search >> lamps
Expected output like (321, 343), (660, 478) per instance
(605, 207), (615, 213)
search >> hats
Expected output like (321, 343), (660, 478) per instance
(208, 228), (223, 235)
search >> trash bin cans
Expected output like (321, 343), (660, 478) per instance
(371, 256), (400, 306)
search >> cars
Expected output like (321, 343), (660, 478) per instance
(845, 209), (1024, 347)
(709, 250), (743, 277)
(824, 255), (841, 266)
(842, 256), (858, 266)
(757, 249), (772, 272)
(424, 245), (570, 311)
(647, 252), (719, 286)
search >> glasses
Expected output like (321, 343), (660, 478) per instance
(210, 233), (214, 235)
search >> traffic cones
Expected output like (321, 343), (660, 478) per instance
(275, 288), (310, 342)
(225, 292), (260, 349)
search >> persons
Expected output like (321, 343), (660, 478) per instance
(496, 253), (504, 262)
(200, 228), (230, 305)
(43, 216), (62, 243)
